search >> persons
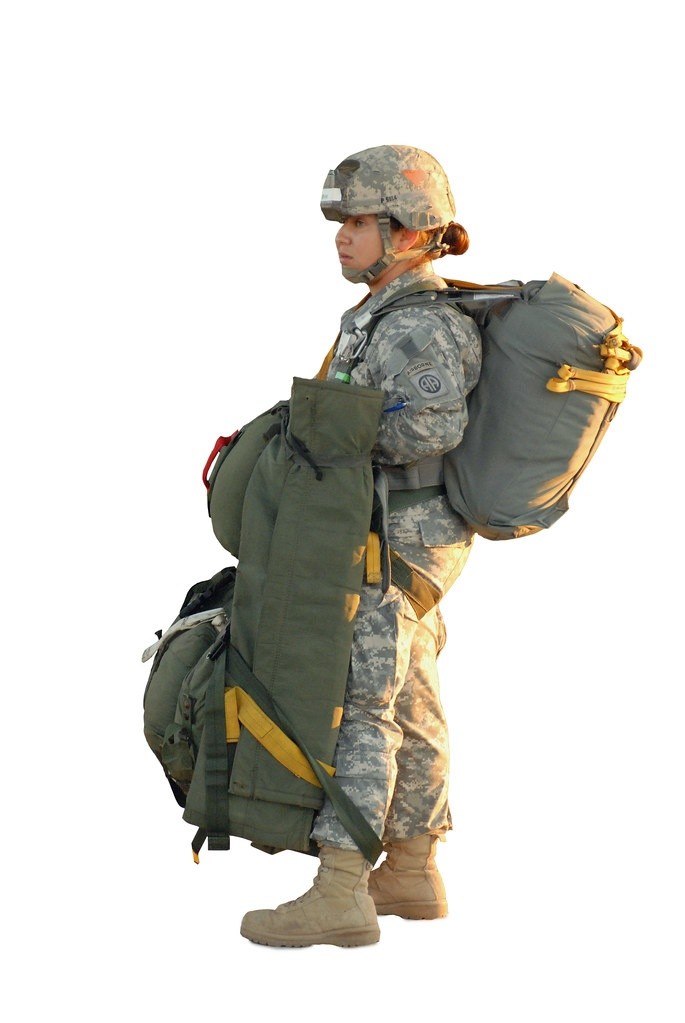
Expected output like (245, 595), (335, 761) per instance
(241, 146), (481, 949)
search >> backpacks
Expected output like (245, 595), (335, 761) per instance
(330, 272), (644, 541)
(543, 541), (544, 550)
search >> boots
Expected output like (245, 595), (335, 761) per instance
(240, 847), (380, 947)
(368, 829), (448, 920)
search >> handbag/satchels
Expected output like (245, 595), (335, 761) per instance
(142, 400), (384, 867)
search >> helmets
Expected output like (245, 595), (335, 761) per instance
(320, 145), (456, 231)
